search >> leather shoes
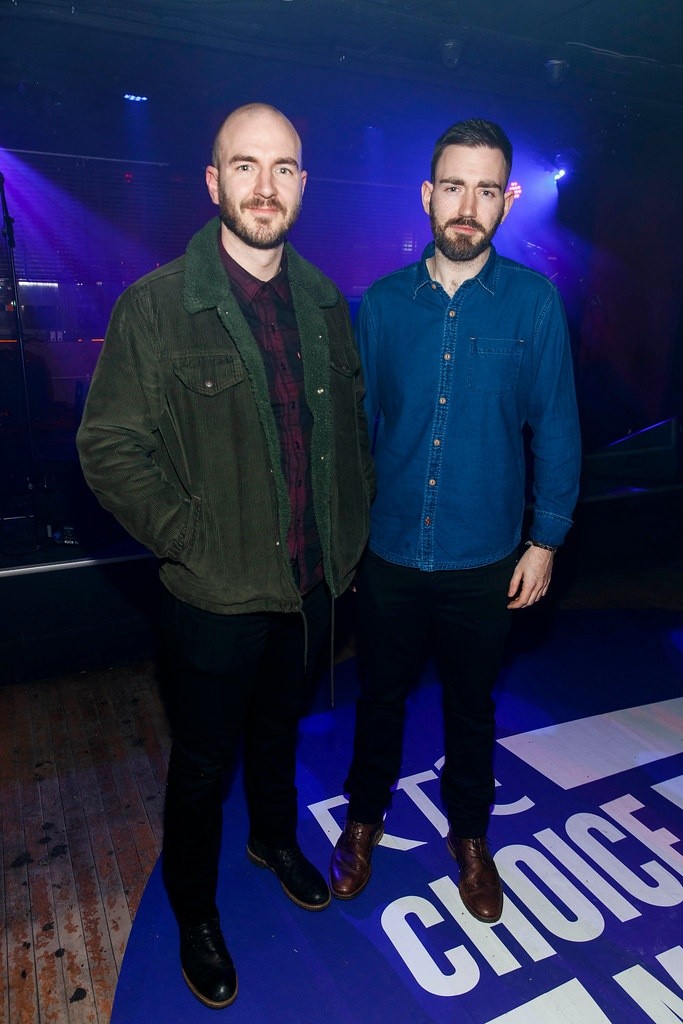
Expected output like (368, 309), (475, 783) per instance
(179, 910), (238, 1010)
(247, 838), (331, 912)
(446, 832), (504, 923)
(329, 817), (384, 900)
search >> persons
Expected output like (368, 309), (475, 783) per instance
(75, 103), (371, 1009)
(328, 116), (583, 923)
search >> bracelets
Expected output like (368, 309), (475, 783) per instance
(525, 540), (559, 554)
